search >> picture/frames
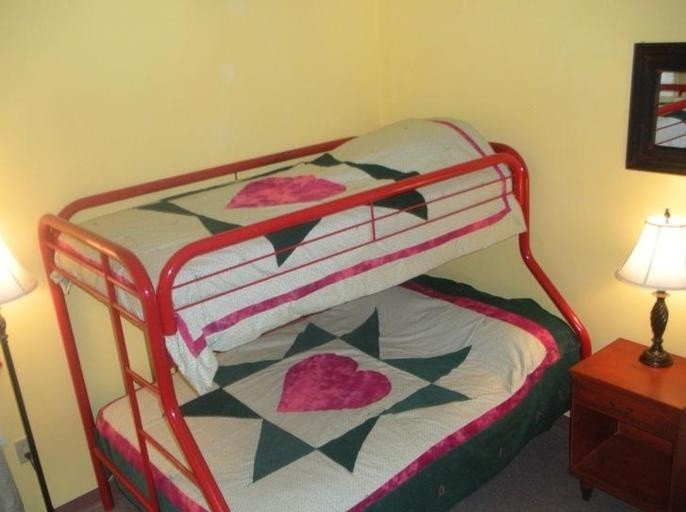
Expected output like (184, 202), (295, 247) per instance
(625, 42), (686, 176)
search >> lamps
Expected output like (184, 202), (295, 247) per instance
(0, 236), (55, 512)
(615, 207), (686, 367)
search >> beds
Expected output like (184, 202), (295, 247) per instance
(38, 116), (591, 512)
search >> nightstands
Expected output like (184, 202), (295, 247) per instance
(568, 338), (686, 512)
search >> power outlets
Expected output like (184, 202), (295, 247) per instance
(15, 438), (33, 464)
